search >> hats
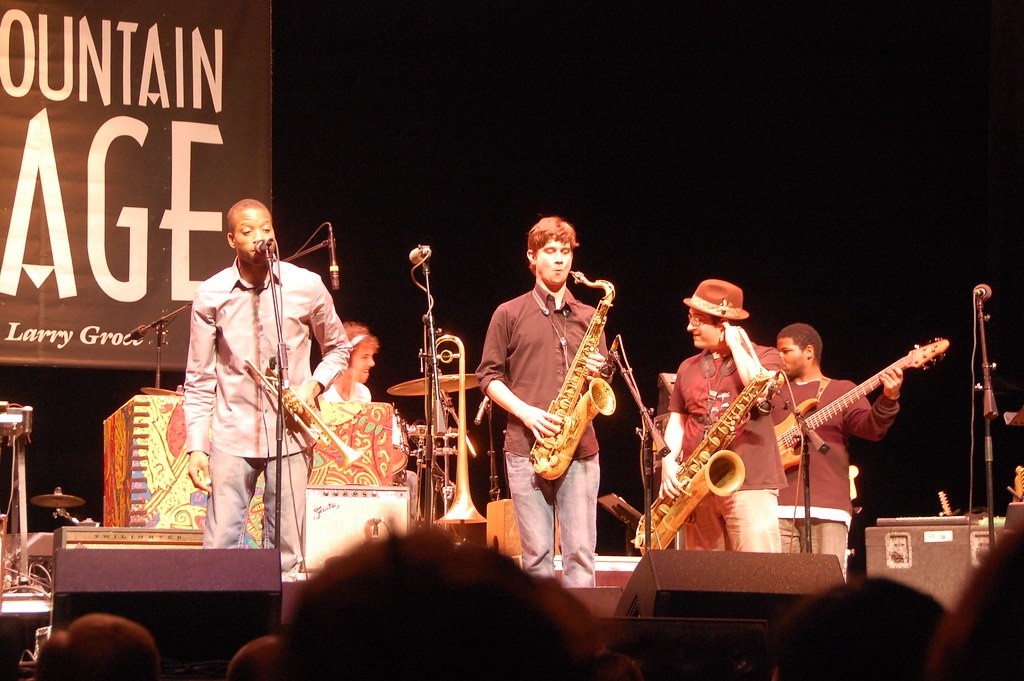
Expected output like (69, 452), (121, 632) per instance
(683, 279), (748, 320)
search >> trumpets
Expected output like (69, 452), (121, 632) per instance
(242, 358), (366, 471)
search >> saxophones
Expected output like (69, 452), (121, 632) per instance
(631, 368), (786, 557)
(530, 270), (617, 481)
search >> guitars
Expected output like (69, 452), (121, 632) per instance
(774, 337), (950, 472)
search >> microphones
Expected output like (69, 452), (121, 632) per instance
(757, 375), (779, 416)
(973, 284), (992, 303)
(254, 238), (275, 253)
(596, 335), (621, 384)
(329, 226), (339, 291)
(408, 245), (432, 265)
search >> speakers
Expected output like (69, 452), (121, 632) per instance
(617, 549), (844, 618)
(52, 549), (282, 662)
(864, 526), (1004, 613)
(305, 484), (408, 572)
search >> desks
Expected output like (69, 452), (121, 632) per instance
(102, 397), (404, 529)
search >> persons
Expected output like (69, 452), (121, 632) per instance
(185, 198), (352, 582)
(655, 276), (903, 592)
(319, 323), (378, 402)
(476, 217), (610, 587)
(30, 504), (1024, 681)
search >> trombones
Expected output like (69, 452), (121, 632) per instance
(433, 333), (489, 523)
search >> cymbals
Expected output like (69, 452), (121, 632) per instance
(141, 386), (185, 396)
(389, 372), (486, 396)
(29, 495), (86, 508)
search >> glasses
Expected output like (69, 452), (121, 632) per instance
(688, 315), (718, 327)
